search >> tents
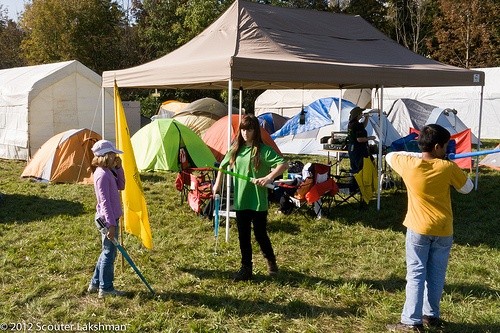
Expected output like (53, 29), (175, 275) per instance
(0, 1), (500, 186)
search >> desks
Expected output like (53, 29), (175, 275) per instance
(317, 150), (387, 190)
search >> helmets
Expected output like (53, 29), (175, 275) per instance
(288, 160), (304, 173)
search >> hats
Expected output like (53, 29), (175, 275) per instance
(91, 140), (123, 155)
(349, 107), (366, 116)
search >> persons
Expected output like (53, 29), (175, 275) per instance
(213, 114), (290, 283)
(86, 139), (126, 299)
(384, 123), (475, 332)
(347, 107), (376, 195)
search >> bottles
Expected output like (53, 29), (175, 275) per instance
(283, 169), (288, 180)
(362, 113), (373, 117)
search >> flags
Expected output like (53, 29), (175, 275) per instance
(114, 83), (154, 250)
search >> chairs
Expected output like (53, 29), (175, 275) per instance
(178, 147), (361, 221)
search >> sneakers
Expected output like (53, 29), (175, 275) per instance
(267, 258), (277, 276)
(88, 285), (99, 293)
(99, 289), (125, 297)
(423, 316), (439, 328)
(386, 321), (423, 333)
(236, 263), (252, 280)
